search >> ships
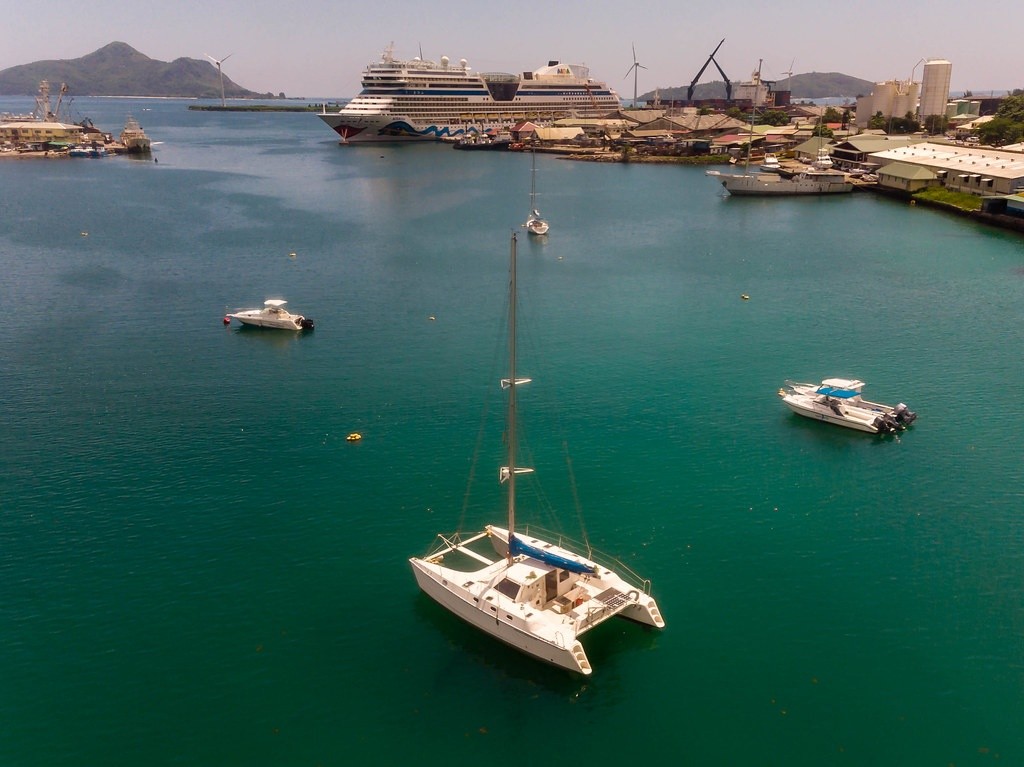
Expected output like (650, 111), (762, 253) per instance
(316, 40), (624, 143)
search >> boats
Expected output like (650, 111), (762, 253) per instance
(226, 299), (317, 333)
(779, 376), (916, 439)
(120, 112), (155, 153)
(66, 142), (108, 158)
(758, 150), (781, 172)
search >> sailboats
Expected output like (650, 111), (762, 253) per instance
(407, 228), (665, 675)
(524, 142), (549, 235)
(704, 58), (853, 195)
